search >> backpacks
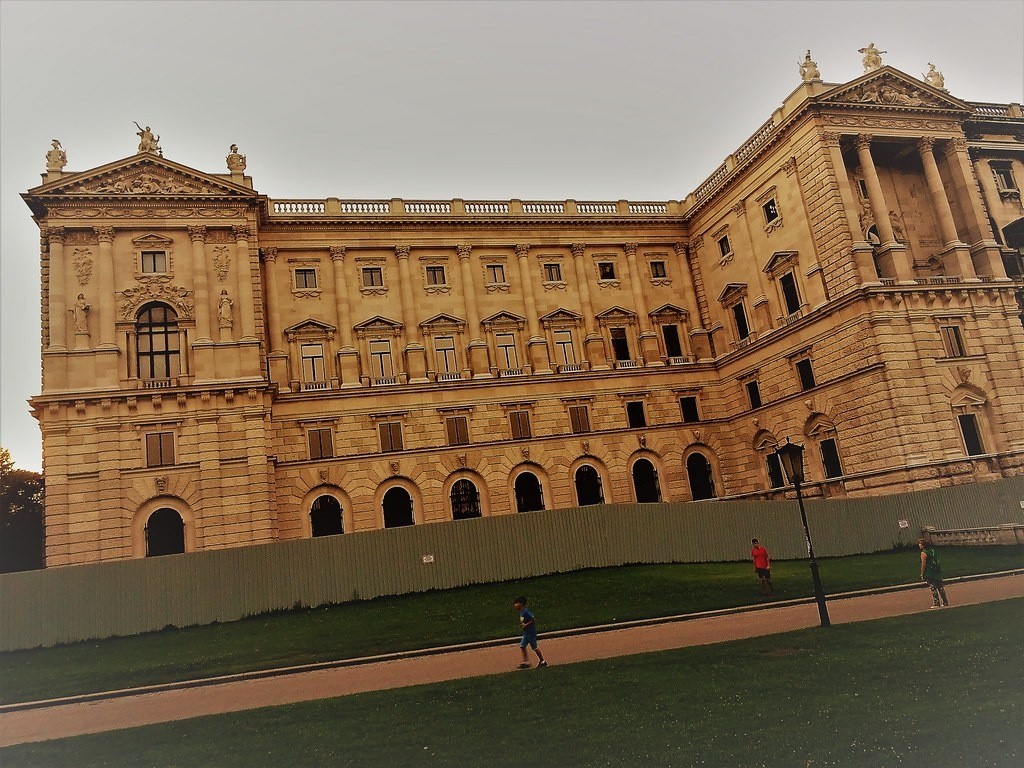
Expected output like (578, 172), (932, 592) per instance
(923, 549), (941, 575)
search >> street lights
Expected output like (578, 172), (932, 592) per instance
(773, 435), (833, 626)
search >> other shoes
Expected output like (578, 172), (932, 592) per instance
(931, 606), (940, 608)
(536, 660), (548, 668)
(940, 604), (945, 607)
(518, 664), (531, 669)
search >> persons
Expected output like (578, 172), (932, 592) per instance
(136, 167), (160, 192)
(860, 85), (882, 104)
(889, 210), (905, 238)
(45, 139), (68, 169)
(900, 87), (923, 106)
(922, 63), (944, 88)
(161, 178), (193, 192)
(917, 539), (948, 609)
(67, 293), (92, 331)
(859, 44), (887, 72)
(751, 539), (774, 596)
(217, 290), (234, 324)
(226, 144), (247, 171)
(798, 50), (821, 79)
(93, 175), (131, 193)
(132, 121), (160, 152)
(880, 79), (900, 104)
(514, 597), (547, 669)
